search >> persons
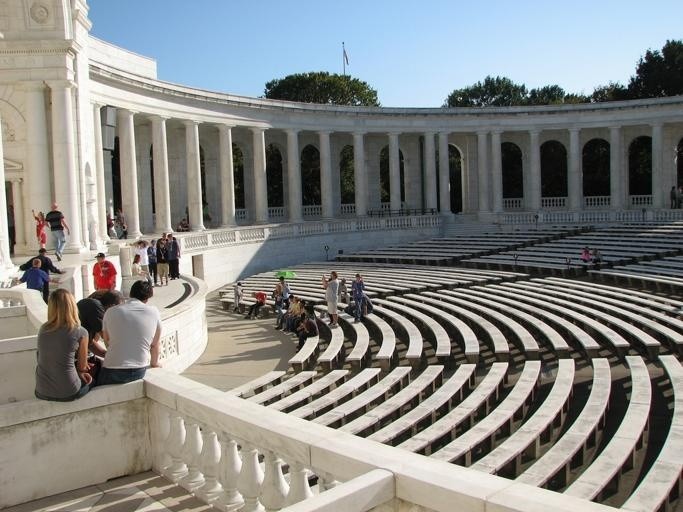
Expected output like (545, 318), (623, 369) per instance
(17, 201), (213, 306)
(76, 290), (126, 358)
(34, 288), (96, 402)
(581, 247), (602, 276)
(233, 271), (373, 352)
(95, 280), (162, 386)
(670, 186), (683, 210)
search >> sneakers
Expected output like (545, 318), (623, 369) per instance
(55, 251), (62, 262)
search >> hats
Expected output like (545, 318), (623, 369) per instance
(39, 248), (48, 254)
(94, 253), (106, 259)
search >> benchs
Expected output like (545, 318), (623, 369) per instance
(219, 227), (683, 510)
